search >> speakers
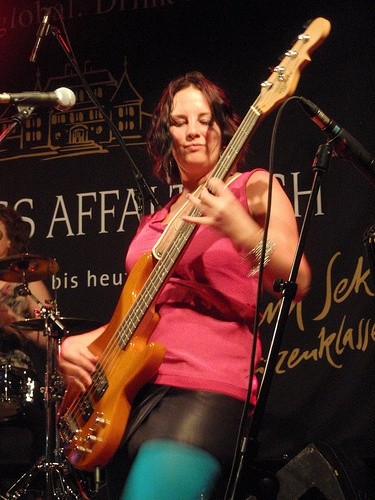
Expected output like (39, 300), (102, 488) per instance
(248, 440), (374, 500)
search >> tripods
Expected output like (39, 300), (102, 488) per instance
(0, 262), (82, 500)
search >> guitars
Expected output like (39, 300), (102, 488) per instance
(60, 18), (333, 470)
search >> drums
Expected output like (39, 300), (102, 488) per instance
(0, 368), (38, 419)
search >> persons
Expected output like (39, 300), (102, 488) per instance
(0, 204), (56, 352)
(57, 68), (310, 500)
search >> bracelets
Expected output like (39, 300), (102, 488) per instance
(243, 232), (278, 278)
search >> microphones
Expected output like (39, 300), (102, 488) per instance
(0, 87), (76, 113)
(29, 9), (51, 62)
(297, 96), (375, 169)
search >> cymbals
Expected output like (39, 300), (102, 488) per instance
(0, 255), (60, 283)
(9, 317), (105, 332)
